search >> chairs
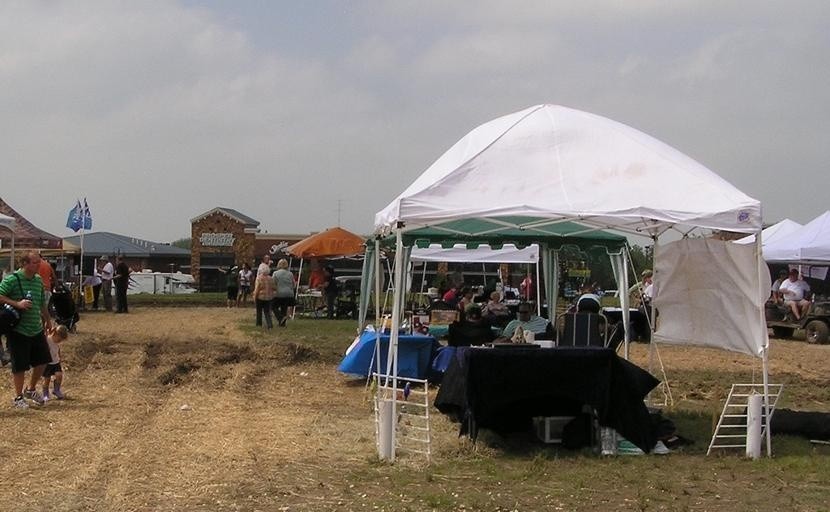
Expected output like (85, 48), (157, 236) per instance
(553, 311), (608, 350)
(607, 322), (626, 353)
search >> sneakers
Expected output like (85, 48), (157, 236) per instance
(41, 390), (50, 402)
(13, 397), (30, 409)
(23, 387), (44, 405)
(52, 389), (64, 399)
(279, 316), (287, 326)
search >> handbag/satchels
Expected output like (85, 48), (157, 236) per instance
(0, 302), (21, 335)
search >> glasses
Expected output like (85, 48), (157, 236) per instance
(518, 310), (531, 314)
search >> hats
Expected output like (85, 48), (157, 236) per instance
(787, 269), (799, 275)
(99, 255), (109, 261)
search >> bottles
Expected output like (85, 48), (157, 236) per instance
(381, 314), (392, 329)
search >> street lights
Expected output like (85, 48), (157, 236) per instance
(644, 246), (650, 264)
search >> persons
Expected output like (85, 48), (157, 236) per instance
(113, 254), (129, 313)
(79, 275), (103, 311)
(0, 247), (54, 409)
(96, 254), (114, 312)
(219, 251), (339, 329)
(47, 278), (80, 333)
(42, 324), (70, 404)
(35, 252), (58, 335)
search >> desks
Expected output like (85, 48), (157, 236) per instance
(433, 344), (664, 462)
(338, 332), (442, 385)
(284, 285), (546, 345)
(604, 304), (645, 343)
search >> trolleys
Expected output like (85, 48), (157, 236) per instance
(49, 282), (81, 335)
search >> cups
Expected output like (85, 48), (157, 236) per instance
(598, 423), (620, 455)
